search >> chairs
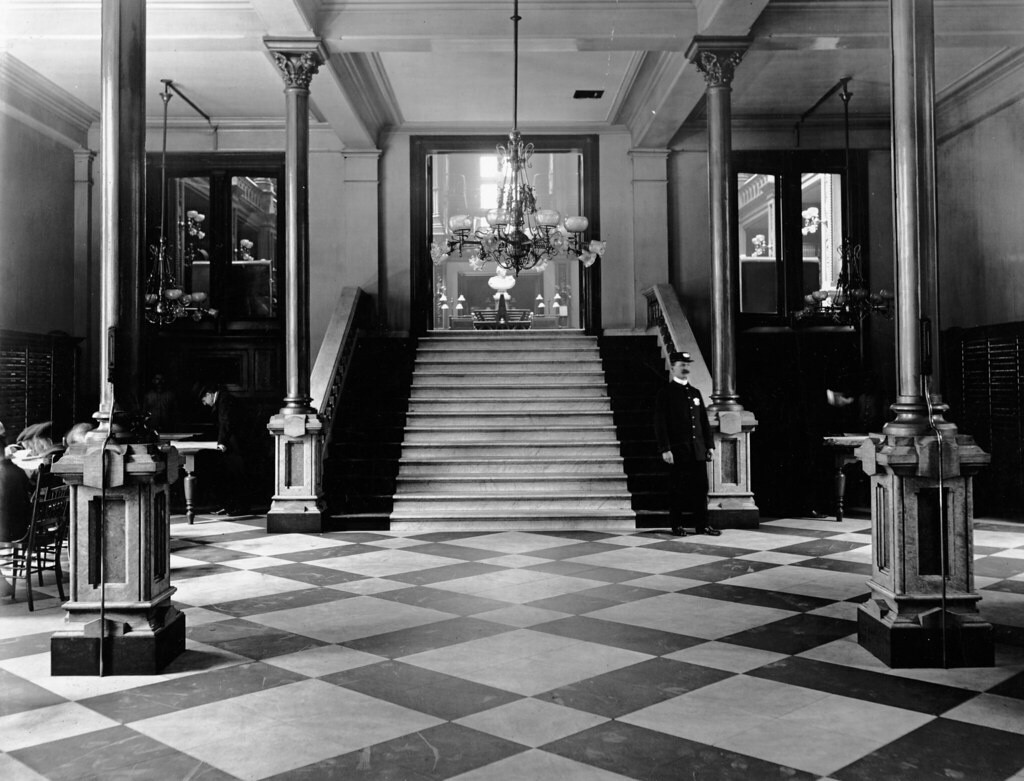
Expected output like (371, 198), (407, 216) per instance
(0, 463), (70, 612)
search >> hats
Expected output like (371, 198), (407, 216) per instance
(670, 352), (694, 364)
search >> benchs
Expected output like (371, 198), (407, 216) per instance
(472, 309), (531, 329)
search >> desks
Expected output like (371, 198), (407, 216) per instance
(823, 437), (871, 522)
(169, 441), (222, 525)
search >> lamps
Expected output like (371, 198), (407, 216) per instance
(794, 76), (895, 326)
(185, 209), (206, 240)
(143, 80), (219, 324)
(429, 0), (606, 278)
(750, 235), (774, 257)
(235, 238), (254, 261)
(801, 207), (829, 235)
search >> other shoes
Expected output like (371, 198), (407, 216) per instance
(0, 583), (13, 597)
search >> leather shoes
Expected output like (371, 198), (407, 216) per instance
(696, 525), (721, 536)
(673, 527), (687, 536)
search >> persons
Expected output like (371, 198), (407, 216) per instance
(655, 352), (722, 537)
(191, 380), (260, 517)
(0, 434), (29, 597)
(145, 373), (171, 408)
